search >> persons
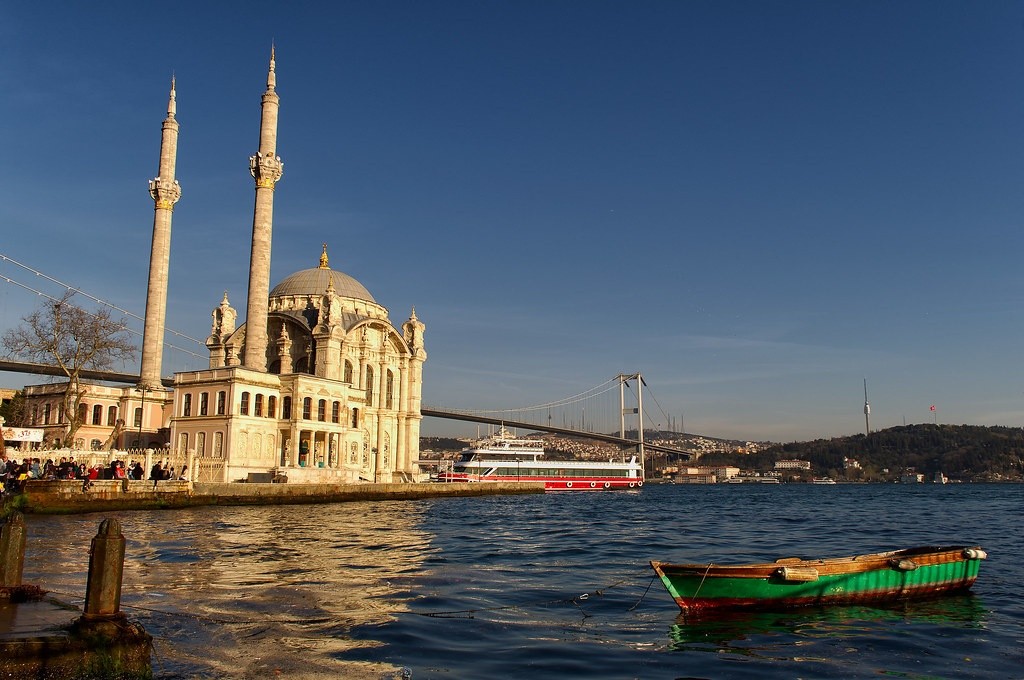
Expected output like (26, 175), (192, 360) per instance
(0, 452), (189, 493)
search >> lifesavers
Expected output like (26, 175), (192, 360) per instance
(637, 480), (643, 487)
(566, 481), (573, 488)
(629, 482), (634, 488)
(604, 481), (611, 489)
(590, 481), (596, 488)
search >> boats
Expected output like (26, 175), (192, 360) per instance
(729, 479), (743, 484)
(436, 420), (644, 492)
(761, 478), (780, 484)
(650, 543), (986, 612)
(812, 477), (837, 485)
(668, 590), (992, 653)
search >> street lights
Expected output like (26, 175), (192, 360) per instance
(134, 383), (152, 452)
